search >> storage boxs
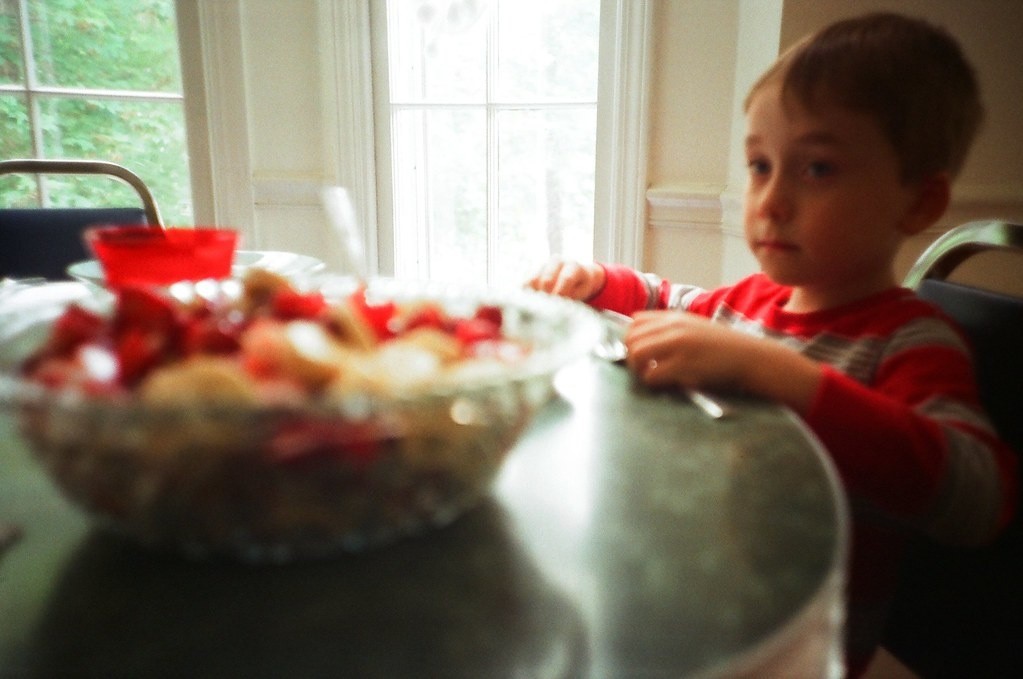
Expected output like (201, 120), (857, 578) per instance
(0, 157), (172, 279)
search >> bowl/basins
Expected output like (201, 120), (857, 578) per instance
(86, 226), (237, 292)
(1, 307), (564, 564)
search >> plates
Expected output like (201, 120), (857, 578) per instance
(68, 249), (327, 293)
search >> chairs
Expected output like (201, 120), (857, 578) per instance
(900, 220), (1023, 448)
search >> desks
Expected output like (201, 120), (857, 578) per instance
(0, 265), (855, 678)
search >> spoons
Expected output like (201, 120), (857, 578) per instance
(607, 308), (735, 420)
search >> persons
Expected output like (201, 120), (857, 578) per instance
(520, 11), (1016, 679)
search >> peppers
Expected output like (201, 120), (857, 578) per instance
(23, 265), (509, 465)
